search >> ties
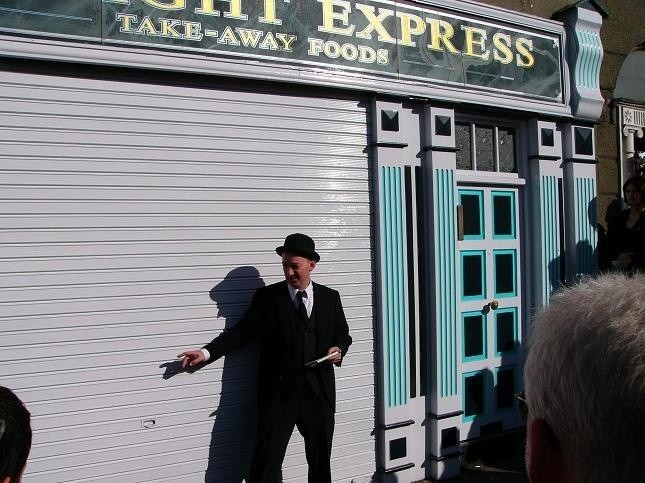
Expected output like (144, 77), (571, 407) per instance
(296, 291), (308, 329)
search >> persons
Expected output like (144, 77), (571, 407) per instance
(0, 386), (34, 482)
(512, 272), (645, 483)
(598, 173), (644, 277)
(175, 232), (352, 483)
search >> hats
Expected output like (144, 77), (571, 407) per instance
(276, 234), (320, 263)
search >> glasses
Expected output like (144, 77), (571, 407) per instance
(514, 390), (529, 420)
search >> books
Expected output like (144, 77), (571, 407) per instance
(305, 351), (339, 368)
